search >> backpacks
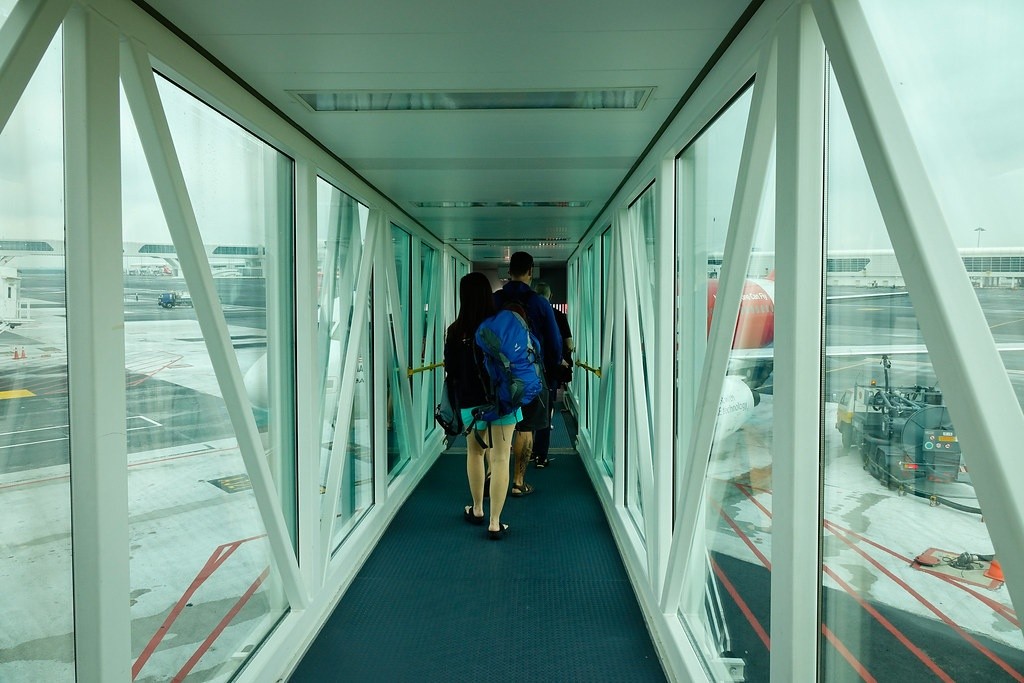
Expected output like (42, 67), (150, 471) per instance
(463, 309), (546, 449)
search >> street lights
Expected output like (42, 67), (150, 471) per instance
(974, 227), (987, 247)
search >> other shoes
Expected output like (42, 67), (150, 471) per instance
(534, 456), (548, 468)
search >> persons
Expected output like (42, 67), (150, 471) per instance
(444, 272), (523, 541)
(491, 251), (576, 497)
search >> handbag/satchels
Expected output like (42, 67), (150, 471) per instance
(435, 377), (462, 436)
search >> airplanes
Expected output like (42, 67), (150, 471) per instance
(706, 271), (1024, 446)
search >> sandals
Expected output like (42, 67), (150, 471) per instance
(511, 481), (535, 496)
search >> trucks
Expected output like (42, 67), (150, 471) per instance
(157, 292), (192, 309)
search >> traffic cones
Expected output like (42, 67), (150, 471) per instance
(12, 347), (21, 359)
(20, 346), (27, 358)
(984, 556), (1005, 582)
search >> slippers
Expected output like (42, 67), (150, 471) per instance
(464, 506), (485, 524)
(488, 522), (509, 539)
